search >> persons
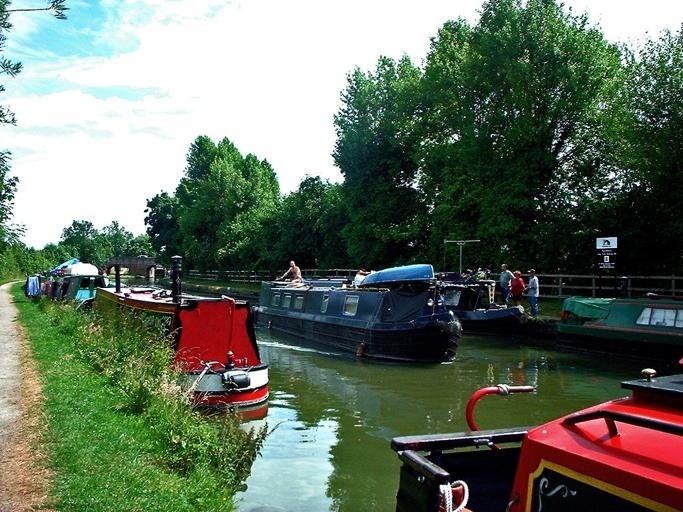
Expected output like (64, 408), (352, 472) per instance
(353, 265), (370, 284)
(510, 269), (525, 306)
(285, 260), (302, 288)
(48, 274), (58, 302)
(498, 263), (512, 304)
(525, 268), (540, 316)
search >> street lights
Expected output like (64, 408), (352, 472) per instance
(443, 238), (481, 274)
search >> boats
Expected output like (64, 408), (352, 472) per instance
(553, 294), (682, 366)
(249, 263), (462, 365)
(390, 358), (681, 512)
(91, 256), (268, 409)
(437, 279), (524, 337)
(39, 258), (125, 309)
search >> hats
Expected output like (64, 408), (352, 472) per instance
(527, 269), (535, 273)
(514, 271), (520, 275)
